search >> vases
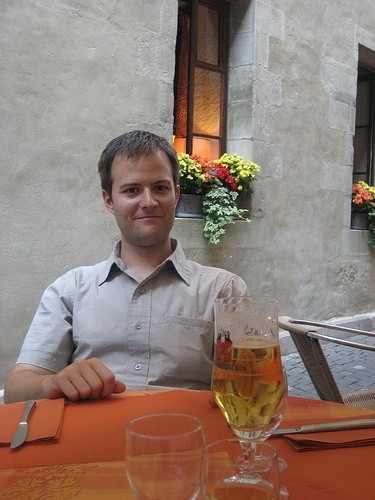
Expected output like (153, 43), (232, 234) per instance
(176, 194), (204, 218)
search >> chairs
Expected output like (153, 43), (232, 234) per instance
(273, 314), (375, 410)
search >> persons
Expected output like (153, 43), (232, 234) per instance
(2, 130), (255, 403)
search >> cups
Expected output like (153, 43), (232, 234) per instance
(125, 413), (208, 500)
(204, 439), (280, 500)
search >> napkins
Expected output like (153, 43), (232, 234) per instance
(0, 398), (64, 445)
(278, 414), (375, 452)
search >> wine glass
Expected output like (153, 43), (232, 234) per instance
(211, 296), (289, 500)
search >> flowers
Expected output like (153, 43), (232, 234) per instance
(350, 181), (375, 247)
(351, 212), (369, 230)
(177, 151), (261, 246)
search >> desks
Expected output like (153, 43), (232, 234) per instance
(0, 392), (375, 500)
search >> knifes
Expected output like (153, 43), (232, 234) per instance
(273, 419), (375, 433)
(10, 400), (36, 448)
